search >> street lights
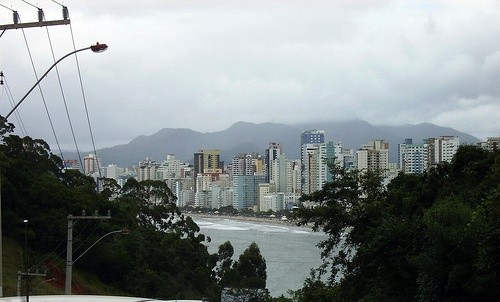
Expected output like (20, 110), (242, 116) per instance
(64, 208), (130, 296)
(22, 219), (29, 302)
